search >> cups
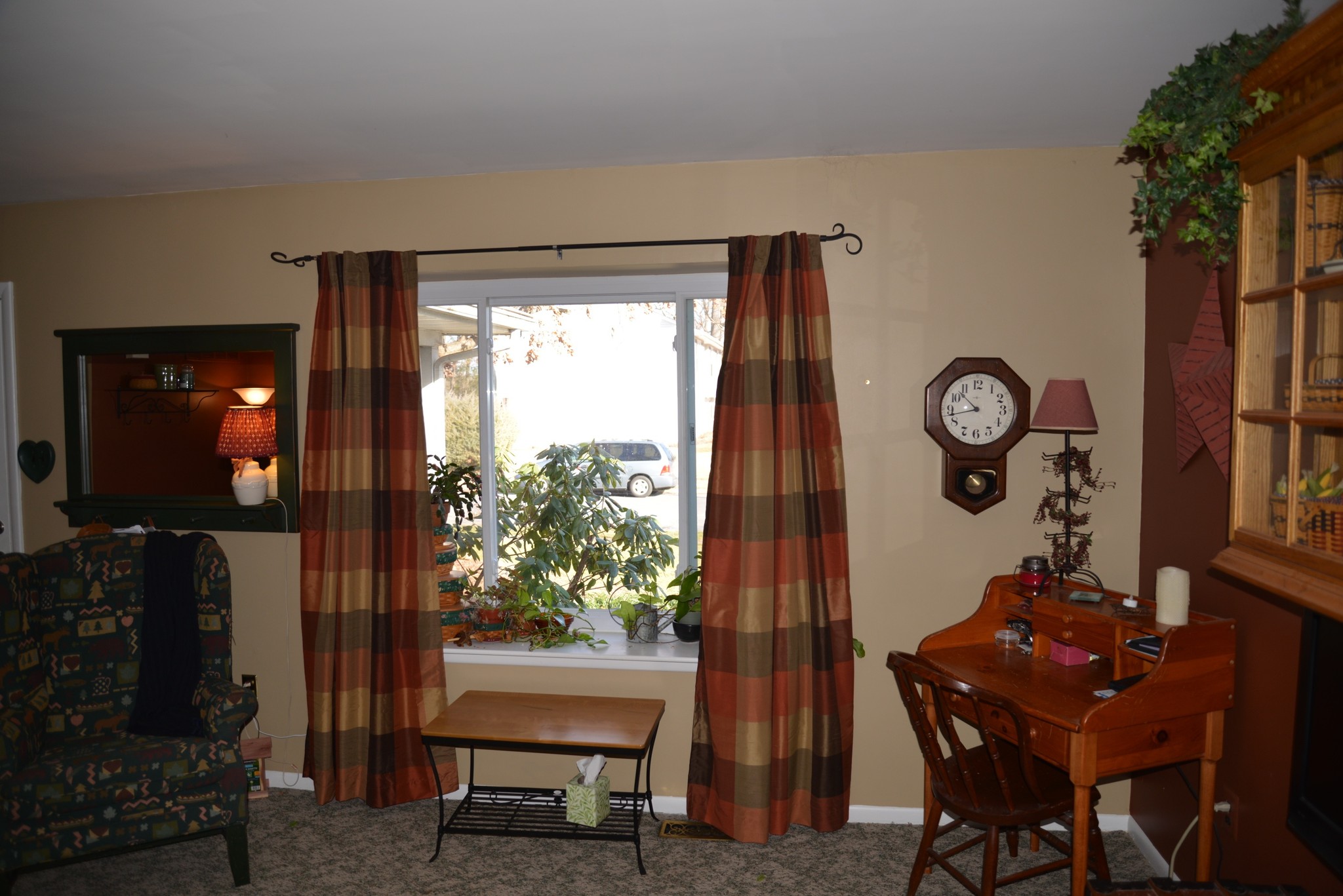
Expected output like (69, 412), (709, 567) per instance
(154, 364), (177, 390)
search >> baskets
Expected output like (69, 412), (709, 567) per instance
(1300, 497), (1343, 557)
(1284, 355), (1343, 412)
(1303, 170), (1343, 269)
(1269, 493), (1301, 535)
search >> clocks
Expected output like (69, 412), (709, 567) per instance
(924, 358), (1030, 516)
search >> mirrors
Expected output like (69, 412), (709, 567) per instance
(53, 324), (301, 534)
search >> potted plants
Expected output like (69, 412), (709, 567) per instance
(613, 580), (660, 644)
(458, 576), (518, 642)
(663, 551), (702, 643)
(504, 589), (608, 652)
(426, 452), (481, 539)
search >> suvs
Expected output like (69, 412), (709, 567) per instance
(498, 440), (676, 498)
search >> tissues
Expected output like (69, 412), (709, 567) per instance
(566, 753), (611, 828)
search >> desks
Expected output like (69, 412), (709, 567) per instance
(421, 691), (665, 876)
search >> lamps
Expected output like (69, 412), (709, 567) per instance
(232, 388), (275, 405)
(264, 406), (278, 499)
(1029, 377), (1111, 598)
(214, 406), (278, 506)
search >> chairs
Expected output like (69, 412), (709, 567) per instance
(886, 650), (1112, 896)
(1, 532), (257, 896)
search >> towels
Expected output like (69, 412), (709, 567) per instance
(127, 529), (217, 736)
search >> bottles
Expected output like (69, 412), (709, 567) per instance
(179, 364), (195, 391)
(127, 371), (158, 390)
(994, 629), (1021, 650)
(1018, 555), (1052, 587)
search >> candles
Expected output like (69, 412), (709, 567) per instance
(1156, 566), (1190, 625)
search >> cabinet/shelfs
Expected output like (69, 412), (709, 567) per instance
(917, 573), (1235, 896)
(1212, 0), (1343, 620)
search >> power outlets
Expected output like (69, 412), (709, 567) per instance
(1223, 786), (1240, 842)
(242, 674), (257, 698)
